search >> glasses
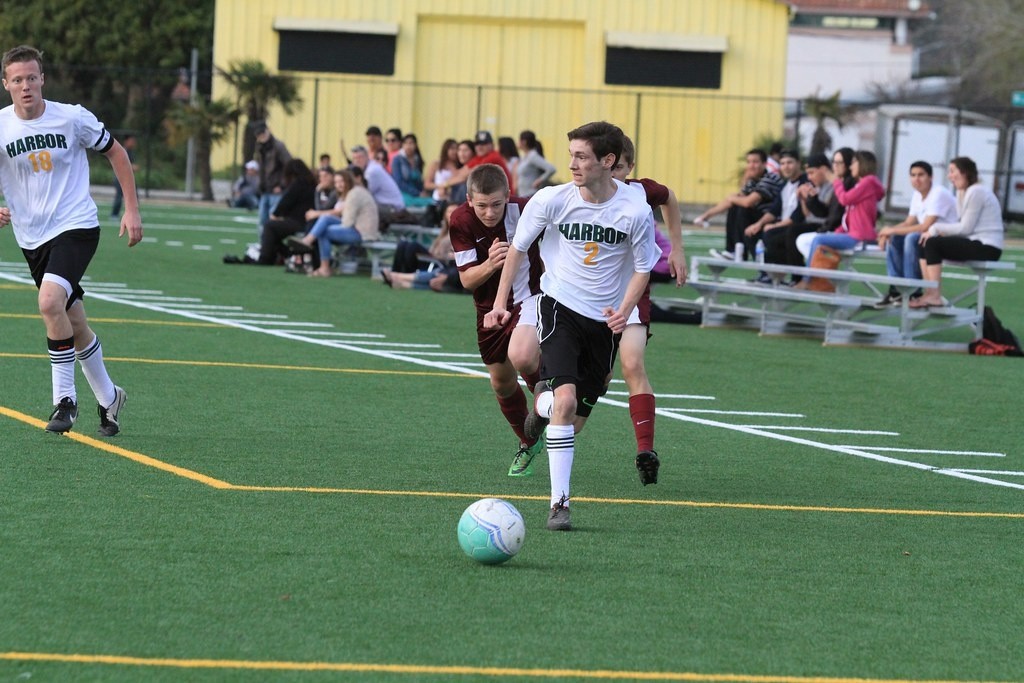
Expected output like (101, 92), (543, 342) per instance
(385, 139), (398, 142)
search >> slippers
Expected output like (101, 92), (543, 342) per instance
(908, 295), (944, 308)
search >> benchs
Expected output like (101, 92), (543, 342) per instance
(689, 239), (1018, 350)
(348, 206), (445, 280)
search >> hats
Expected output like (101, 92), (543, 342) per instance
(253, 121), (265, 134)
(475, 131), (492, 144)
(802, 153), (830, 168)
(245, 160), (259, 170)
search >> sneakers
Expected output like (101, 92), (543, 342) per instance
(635, 450), (660, 486)
(547, 491), (571, 530)
(541, 426), (547, 441)
(96, 384), (127, 437)
(507, 435), (544, 477)
(523, 380), (552, 440)
(45, 396), (79, 435)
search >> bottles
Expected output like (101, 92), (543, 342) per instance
(735, 242), (744, 261)
(755, 239), (765, 262)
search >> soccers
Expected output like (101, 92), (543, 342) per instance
(454, 497), (527, 565)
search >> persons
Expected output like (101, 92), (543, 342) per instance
(0, 45), (143, 436)
(598, 136), (687, 487)
(697, 141), (856, 292)
(449, 164), (553, 478)
(650, 221), (680, 285)
(876, 162), (957, 306)
(112, 132), (141, 219)
(909, 157), (1005, 308)
(799, 151), (885, 290)
(484, 121), (656, 529)
(222, 119), (555, 293)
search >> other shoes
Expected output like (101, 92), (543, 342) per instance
(286, 236), (313, 253)
(877, 285), (901, 305)
(893, 289), (922, 306)
(756, 277), (772, 286)
(711, 248), (733, 259)
(381, 268), (401, 290)
(795, 278), (810, 290)
(747, 275), (762, 283)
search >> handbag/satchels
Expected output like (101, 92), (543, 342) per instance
(810, 246), (841, 293)
(421, 199), (448, 229)
